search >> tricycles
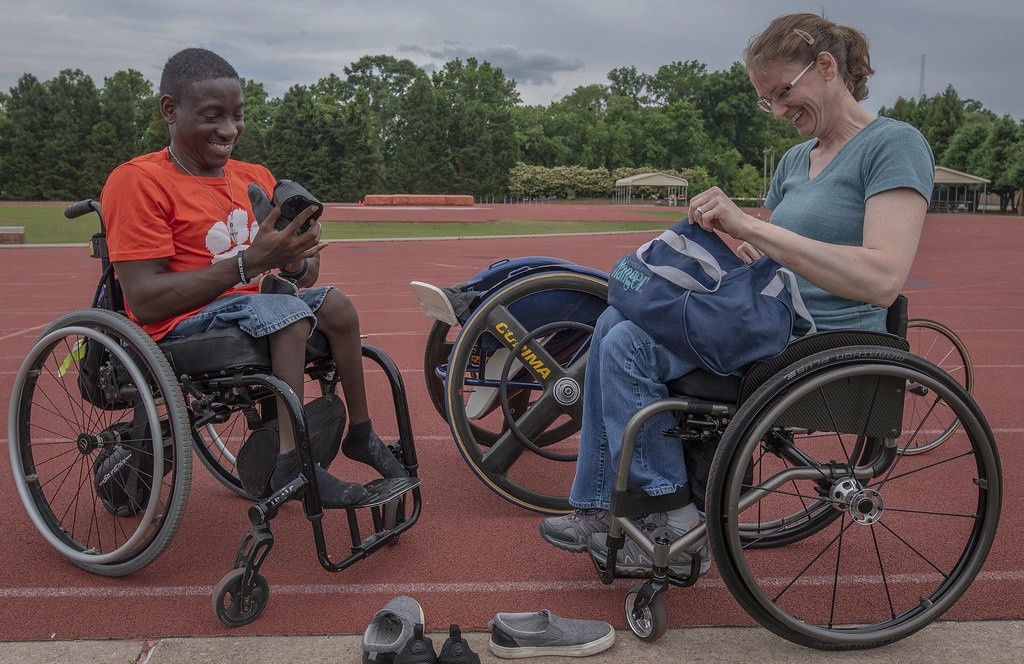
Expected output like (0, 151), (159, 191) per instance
(408, 257), (977, 517)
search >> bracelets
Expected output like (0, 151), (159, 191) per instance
(283, 260), (308, 280)
(237, 251), (250, 285)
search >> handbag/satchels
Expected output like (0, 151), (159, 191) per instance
(236, 393), (347, 498)
(607, 217), (817, 376)
(77, 262), (163, 411)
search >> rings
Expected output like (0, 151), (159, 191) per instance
(697, 207), (704, 214)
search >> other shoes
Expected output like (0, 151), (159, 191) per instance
(394, 623), (437, 664)
(437, 624), (481, 664)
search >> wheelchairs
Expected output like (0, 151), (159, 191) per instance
(6, 197), (423, 629)
(589, 290), (1006, 652)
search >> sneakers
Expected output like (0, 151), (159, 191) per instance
(487, 609), (616, 658)
(586, 511), (711, 579)
(538, 508), (649, 553)
(361, 595), (425, 664)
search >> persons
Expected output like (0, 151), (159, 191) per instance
(102, 47), (408, 504)
(540, 12), (934, 581)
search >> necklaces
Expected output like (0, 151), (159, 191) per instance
(168, 147), (237, 242)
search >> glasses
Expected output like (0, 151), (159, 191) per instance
(756, 58), (818, 113)
